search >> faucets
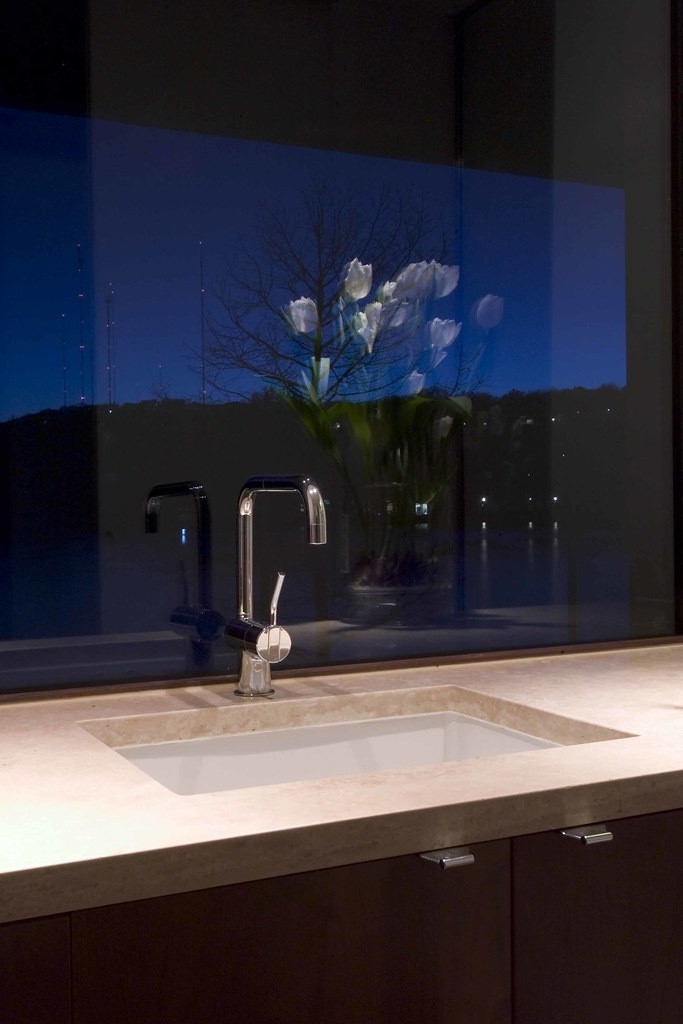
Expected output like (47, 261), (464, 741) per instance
(144, 479), (227, 668)
(223, 475), (328, 698)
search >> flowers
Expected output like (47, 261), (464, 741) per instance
(274, 256), (480, 592)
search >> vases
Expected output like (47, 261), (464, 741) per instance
(332, 481), (466, 626)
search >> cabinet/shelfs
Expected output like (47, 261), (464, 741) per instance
(0, 646), (683, 1024)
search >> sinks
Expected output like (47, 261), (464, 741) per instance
(1, 637), (318, 694)
(75, 685), (641, 796)
